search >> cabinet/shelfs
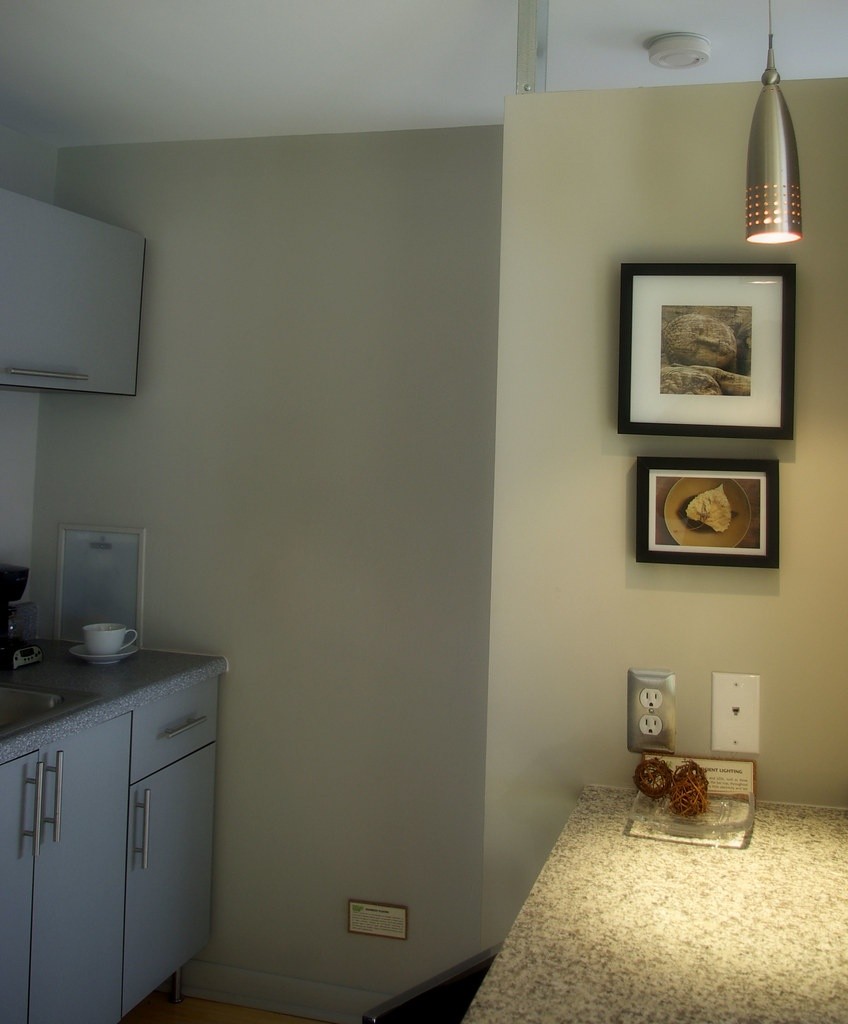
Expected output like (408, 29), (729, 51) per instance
(0, 188), (147, 395)
(0, 639), (227, 1024)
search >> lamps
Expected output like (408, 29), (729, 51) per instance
(745, 0), (802, 245)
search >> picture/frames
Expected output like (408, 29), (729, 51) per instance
(635, 456), (780, 569)
(617, 263), (797, 441)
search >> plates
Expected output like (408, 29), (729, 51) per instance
(68, 643), (139, 664)
(663, 478), (751, 547)
(627, 790), (756, 834)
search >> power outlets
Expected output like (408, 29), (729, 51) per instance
(625, 668), (677, 754)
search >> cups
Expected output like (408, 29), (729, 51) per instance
(82, 624), (138, 655)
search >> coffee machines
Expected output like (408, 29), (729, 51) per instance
(0, 563), (43, 672)
